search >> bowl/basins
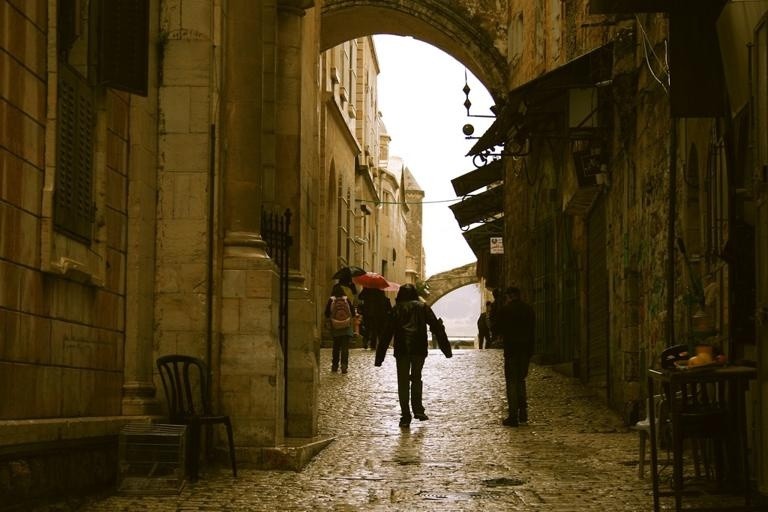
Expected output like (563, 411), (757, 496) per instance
(672, 359), (717, 374)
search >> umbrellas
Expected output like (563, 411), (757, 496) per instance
(332, 266), (366, 281)
(354, 272), (390, 289)
(381, 280), (401, 292)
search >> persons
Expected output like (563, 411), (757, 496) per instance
(501, 286), (537, 426)
(477, 289), (502, 349)
(323, 284), (355, 373)
(370, 292), (392, 346)
(358, 286), (378, 348)
(333, 276), (356, 317)
(374, 283), (452, 427)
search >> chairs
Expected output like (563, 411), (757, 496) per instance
(157, 354), (236, 484)
(637, 343), (767, 512)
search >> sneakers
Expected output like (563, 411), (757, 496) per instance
(398, 412), (429, 428)
(331, 365), (349, 374)
(502, 410), (529, 427)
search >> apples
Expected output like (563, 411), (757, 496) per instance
(687, 353), (711, 366)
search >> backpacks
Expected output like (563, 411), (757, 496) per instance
(327, 295), (351, 330)
